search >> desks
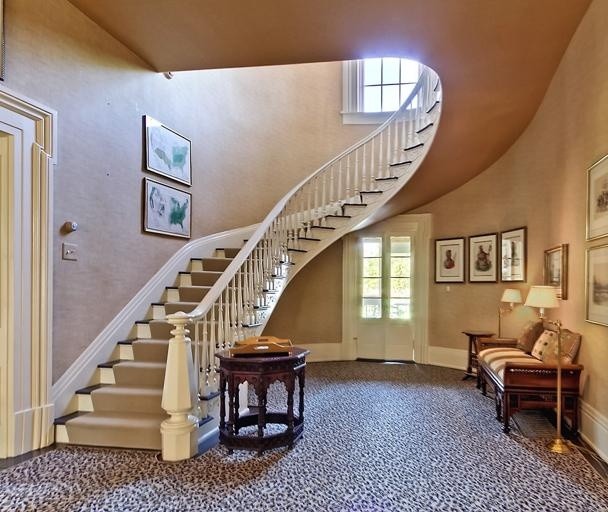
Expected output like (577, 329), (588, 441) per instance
(214, 345), (310, 456)
(461, 329), (495, 382)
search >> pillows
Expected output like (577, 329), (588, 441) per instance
(529, 329), (556, 360)
(540, 329), (581, 367)
(515, 318), (541, 353)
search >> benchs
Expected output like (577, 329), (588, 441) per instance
(475, 336), (580, 435)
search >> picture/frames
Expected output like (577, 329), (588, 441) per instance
(542, 243), (568, 302)
(141, 114), (194, 187)
(433, 237), (465, 284)
(583, 245), (608, 327)
(141, 176), (192, 242)
(467, 232), (499, 284)
(499, 226), (527, 284)
(585, 154), (608, 242)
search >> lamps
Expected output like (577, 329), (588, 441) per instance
(497, 288), (522, 338)
(523, 285), (570, 454)
